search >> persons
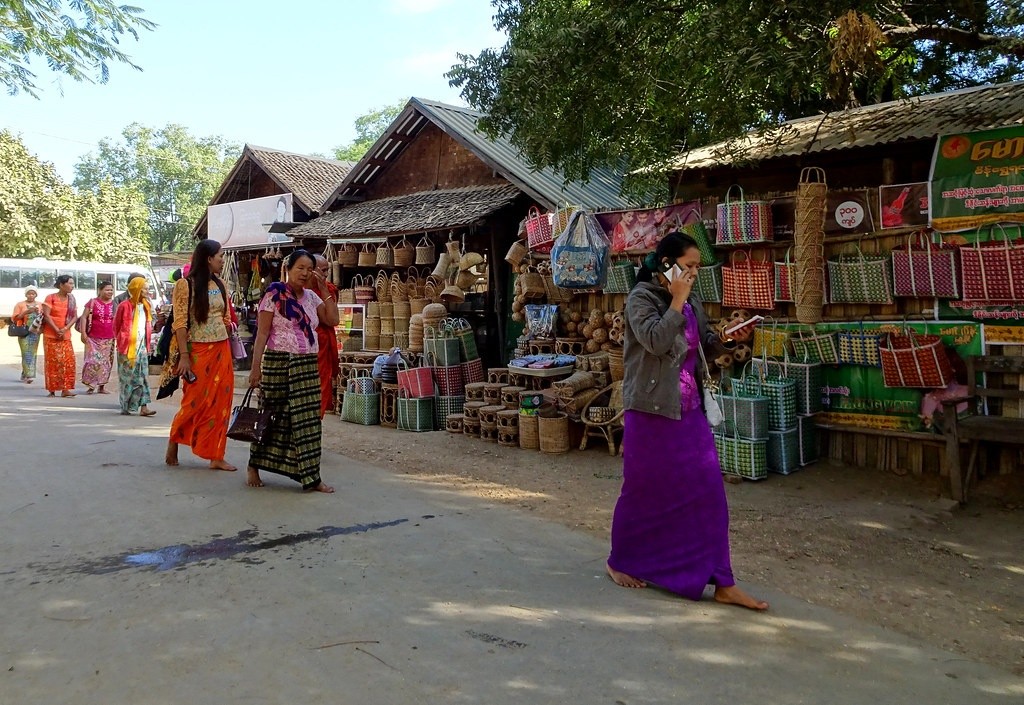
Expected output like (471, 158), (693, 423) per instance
(81, 281), (118, 394)
(113, 272), (156, 330)
(22, 272), (37, 286)
(303, 254), (340, 421)
(605, 232), (769, 611)
(114, 277), (157, 416)
(156, 239), (238, 471)
(11, 284), (44, 384)
(9, 273), (18, 286)
(41, 274), (77, 398)
(267, 196), (290, 242)
(247, 248), (335, 493)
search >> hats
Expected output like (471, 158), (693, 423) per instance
(24, 285), (38, 297)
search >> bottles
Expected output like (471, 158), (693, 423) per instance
(886, 187), (910, 220)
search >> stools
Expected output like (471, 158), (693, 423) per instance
(379, 382), (400, 427)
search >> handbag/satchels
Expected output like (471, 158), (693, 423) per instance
(879, 314), (952, 388)
(9, 314), (28, 336)
(76, 299), (94, 333)
(321, 234), (486, 435)
(226, 385), (275, 446)
(158, 310), (177, 360)
(840, 314), (881, 366)
(891, 227), (960, 299)
(828, 232), (895, 305)
(28, 310), (44, 334)
(678, 168), (840, 480)
(230, 321), (248, 359)
(962, 221), (1023, 304)
(219, 246), (283, 336)
(504, 200), (639, 339)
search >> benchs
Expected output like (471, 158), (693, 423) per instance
(937, 354), (1024, 508)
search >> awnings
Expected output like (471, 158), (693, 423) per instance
(286, 184), (523, 238)
(621, 83), (1024, 177)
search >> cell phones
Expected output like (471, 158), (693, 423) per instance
(658, 257), (690, 285)
(725, 315), (765, 336)
(184, 370), (197, 384)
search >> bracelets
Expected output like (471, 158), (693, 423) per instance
(324, 295), (332, 303)
(180, 351), (191, 355)
(719, 325), (732, 343)
(64, 327), (67, 331)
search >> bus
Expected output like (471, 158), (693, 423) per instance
(0, 257), (170, 319)
